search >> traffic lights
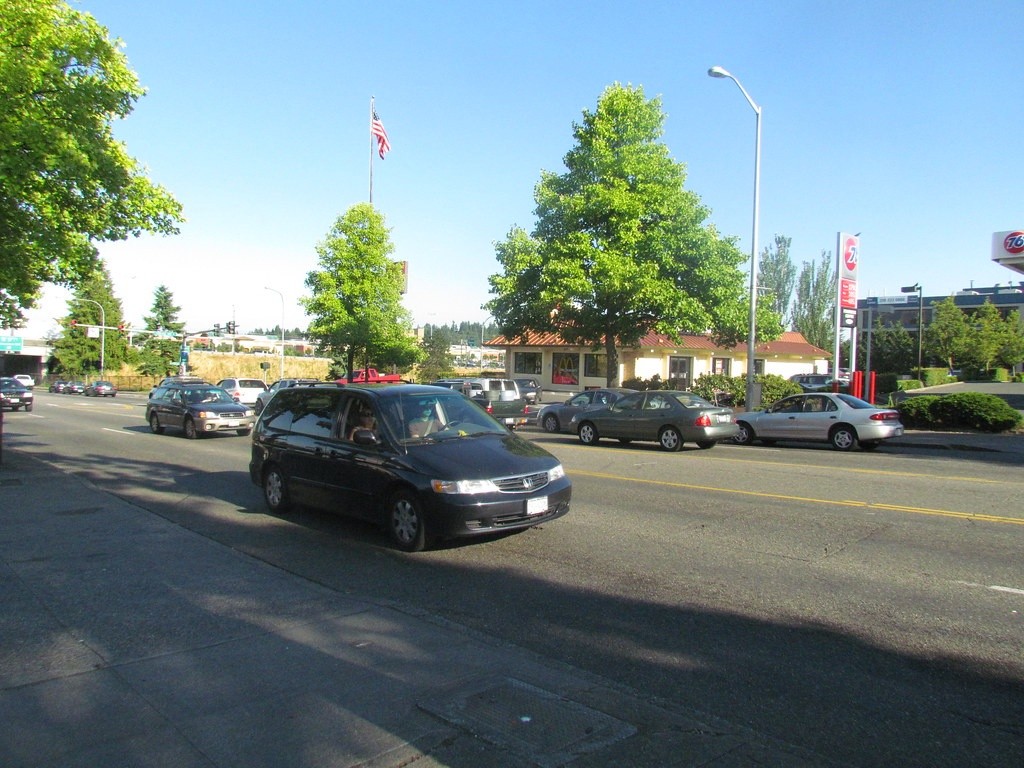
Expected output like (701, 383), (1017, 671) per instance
(118, 324), (125, 335)
(71, 319), (77, 330)
(228, 321), (235, 334)
(214, 323), (220, 337)
(265, 286), (285, 378)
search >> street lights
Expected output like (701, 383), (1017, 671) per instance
(708, 65), (763, 410)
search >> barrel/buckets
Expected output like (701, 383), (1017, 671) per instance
(499, 390), (515, 402)
(482, 390), (501, 401)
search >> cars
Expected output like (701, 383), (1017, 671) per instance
(733, 393), (904, 451)
(49, 381), (68, 393)
(145, 385), (257, 440)
(148, 376), (205, 400)
(433, 378), (543, 405)
(536, 388), (641, 434)
(0, 377), (33, 412)
(63, 380), (87, 395)
(568, 390), (740, 452)
(84, 381), (118, 397)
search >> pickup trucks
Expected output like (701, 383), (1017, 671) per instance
(335, 368), (400, 384)
(14, 375), (35, 390)
(432, 382), (529, 433)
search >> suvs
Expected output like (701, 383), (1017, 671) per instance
(255, 377), (323, 416)
(215, 378), (269, 405)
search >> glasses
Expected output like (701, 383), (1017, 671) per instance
(360, 413), (376, 418)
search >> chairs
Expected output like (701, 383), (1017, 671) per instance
(805, 403), (812, 412)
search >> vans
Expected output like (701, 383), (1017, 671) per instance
(249, 380), (573, 552)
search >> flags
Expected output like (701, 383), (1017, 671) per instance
(372, 109), (391, 159)
